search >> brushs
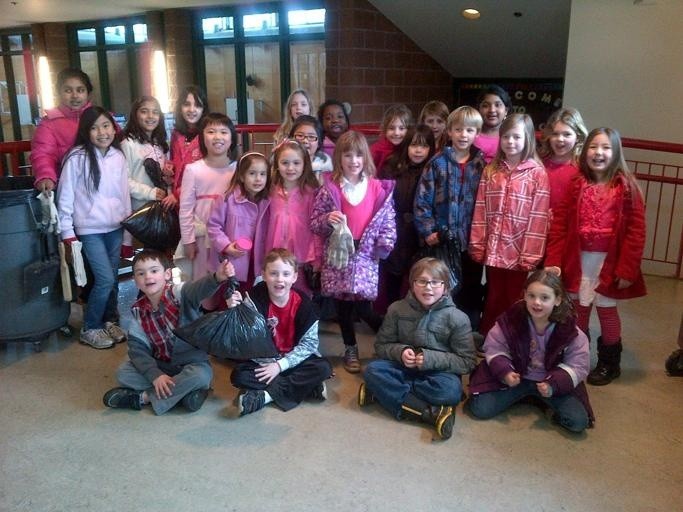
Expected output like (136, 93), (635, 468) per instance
(58, 242), (78, 302)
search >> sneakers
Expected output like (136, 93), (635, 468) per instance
(343, 343), (361, 373)
(79, 327), (115, 349)
(103, 322), (126, 343)
(238, 389), (265, 415)
(182, 389), (209, 412)
(311, 381), (327, 401)
(102, 387), (151, 410)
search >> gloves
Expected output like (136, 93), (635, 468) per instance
(70, 240), (87, 289)
(327, 214), (355, 270)
(36, 191), (62, 235)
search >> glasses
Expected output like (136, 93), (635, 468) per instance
(413, 279), (444, 288)
(291, 135), (319, 141)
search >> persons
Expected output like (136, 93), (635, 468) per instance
(101, 249), (237, 414)
(467, 270), (596, 434)
(32, 68), (645, 387)
(358, 257), (476, 440)
(225, 246), (333, 415)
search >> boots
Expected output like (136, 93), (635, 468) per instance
(359, 382), (378, 406)
(587, 336), (622, 386)
(527, 396), (554, 421)
(401, 393), (455, 439)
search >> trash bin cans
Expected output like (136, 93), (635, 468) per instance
(0, 175), (71, 353)
